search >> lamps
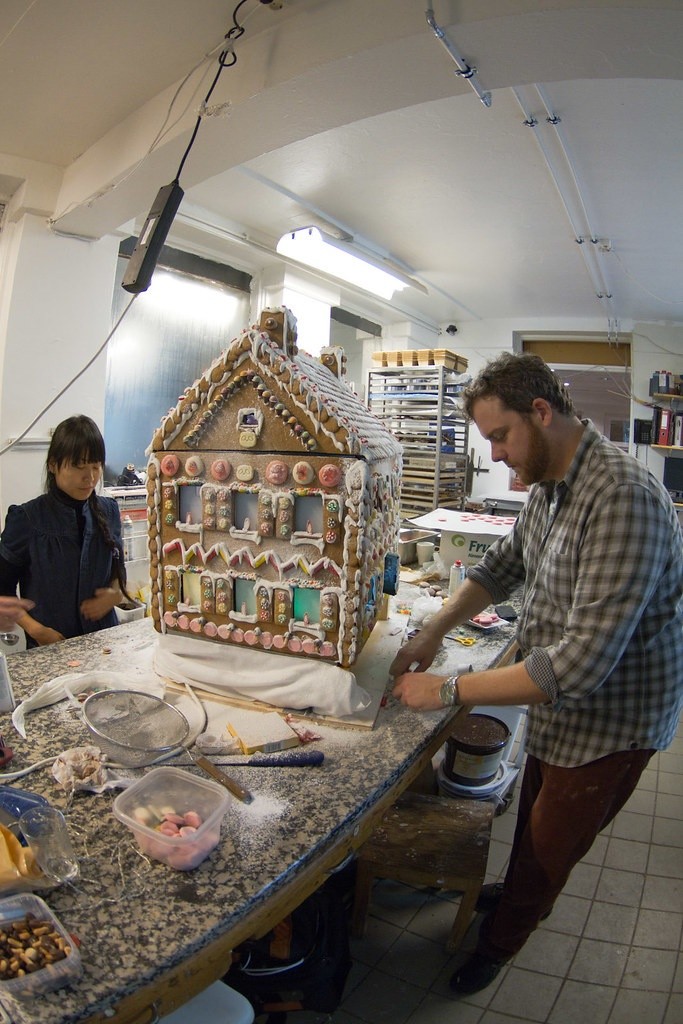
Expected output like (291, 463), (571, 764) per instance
(277, 226), (427, 291)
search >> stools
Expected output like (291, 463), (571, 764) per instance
(357, 796), (496, 945)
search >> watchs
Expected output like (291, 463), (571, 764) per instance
(439, 675), (460, 706)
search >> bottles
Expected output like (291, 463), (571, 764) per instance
(449, 560), (465, 597)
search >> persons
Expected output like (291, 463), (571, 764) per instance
(387, 352), (683, 992)
(0, 415), (126, 650)
(0, 596), (35, 633)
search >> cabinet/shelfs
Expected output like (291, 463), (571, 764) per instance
(363, 366), (469, 520)
(650, 393), (683, 512)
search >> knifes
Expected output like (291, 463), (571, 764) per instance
(145, 750), (325, 767)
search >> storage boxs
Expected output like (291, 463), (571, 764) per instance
(2, 506), (518, 1006)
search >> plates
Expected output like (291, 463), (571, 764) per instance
(466, 611), (509, 629)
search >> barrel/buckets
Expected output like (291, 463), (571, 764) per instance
(437, 757), (508, 805)
(444, 713), (510, 786)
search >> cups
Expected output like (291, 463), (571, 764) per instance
(19, 806), (79, 882)
(416, 541), (440, 565)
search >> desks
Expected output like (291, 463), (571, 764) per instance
(1, 569), (537, 1024)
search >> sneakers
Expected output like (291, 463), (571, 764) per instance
(452, 954), (512, 994)
(474, 883), (553, 921)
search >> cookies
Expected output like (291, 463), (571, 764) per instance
(0, 913), (81, 999)
(143, 308), (405, 667)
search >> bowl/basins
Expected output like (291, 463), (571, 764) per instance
(0, 786), (48, 846)
(0, 893), (83, 1001)
(113, 767), (229, 871)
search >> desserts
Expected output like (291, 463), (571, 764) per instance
(419, 581), (498, 627)
(438, 513), (516, 525)
(127, 805), (216, 870)
